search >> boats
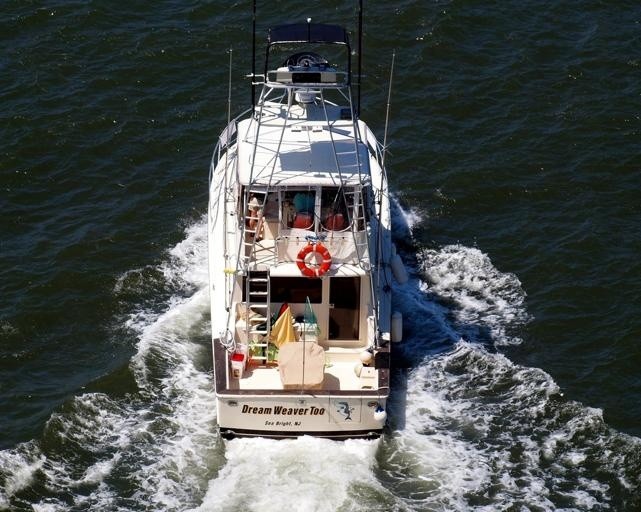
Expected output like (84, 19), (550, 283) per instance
(210, 1), (403, 442)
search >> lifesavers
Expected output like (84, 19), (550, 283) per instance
(296, 243), (332, 277)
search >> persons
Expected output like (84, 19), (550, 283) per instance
(248, 193), (264, 242)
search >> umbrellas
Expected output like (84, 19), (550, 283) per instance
(257, 303), (296, 349)
